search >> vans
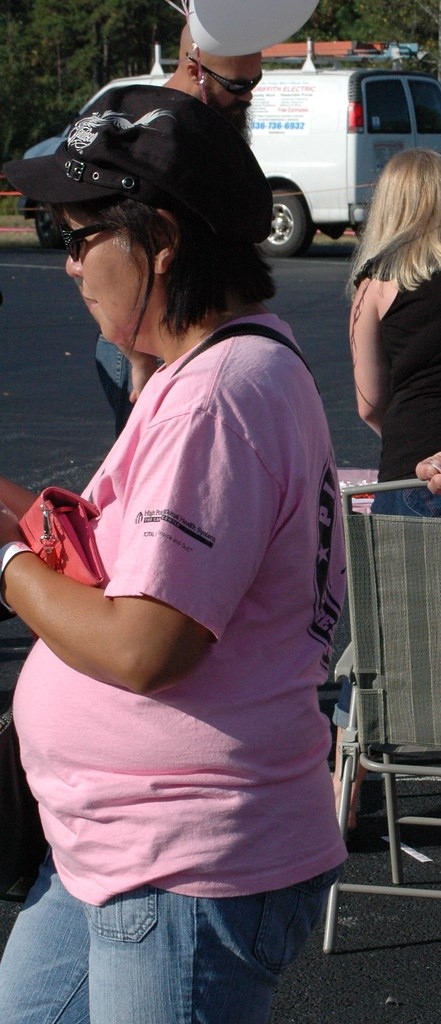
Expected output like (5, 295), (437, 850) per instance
(18, 41), (441, 260)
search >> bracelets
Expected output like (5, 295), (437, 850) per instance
(0, 539), (33, 615)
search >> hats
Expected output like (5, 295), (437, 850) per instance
(2, 85), (272, 244)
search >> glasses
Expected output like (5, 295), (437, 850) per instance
(186, 51), (264, 96)
(47, 206), (112, 261)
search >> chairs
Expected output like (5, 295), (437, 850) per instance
(320, 478), (441, 955)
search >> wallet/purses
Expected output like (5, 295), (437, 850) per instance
(19, 486), (105, 590)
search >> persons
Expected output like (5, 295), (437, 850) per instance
(334, 146), (441, 837)
(0, 82), (352, 1024)
(89, 17), (265, 440)
